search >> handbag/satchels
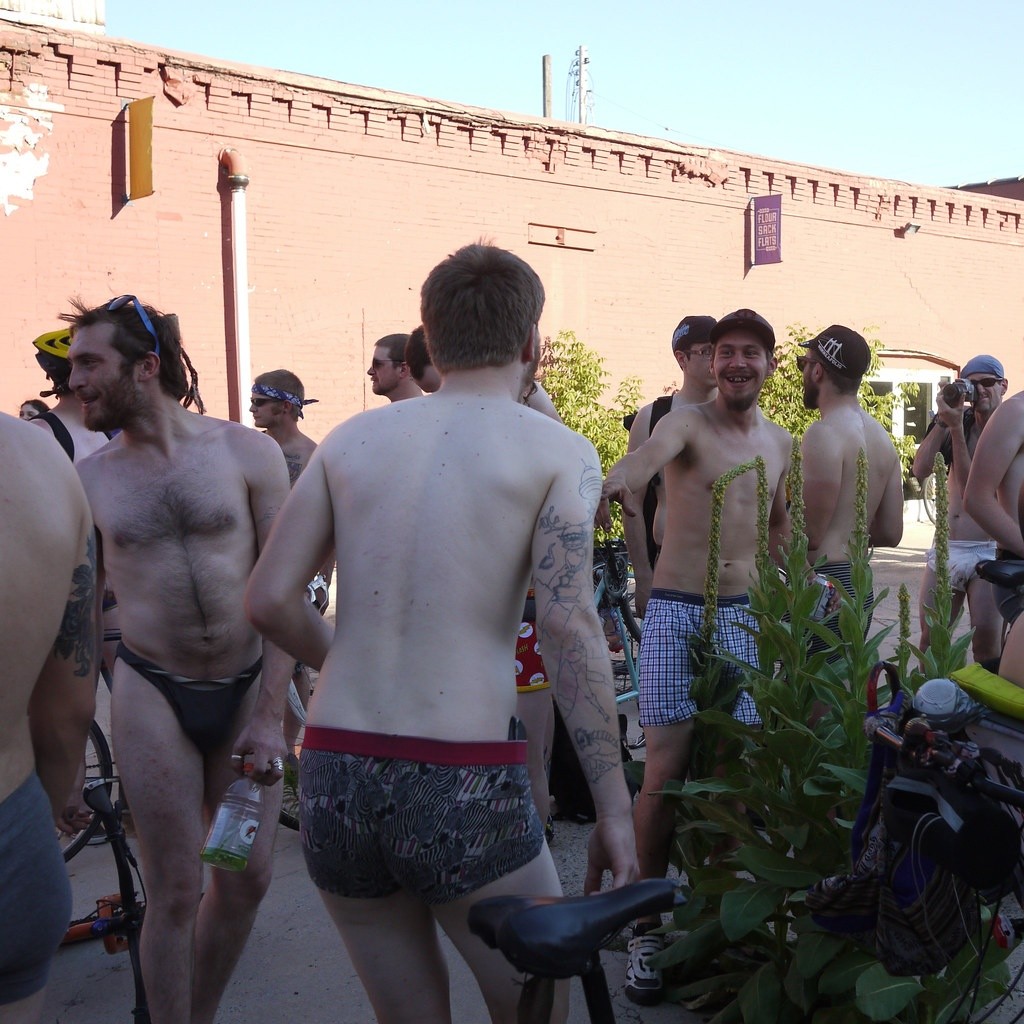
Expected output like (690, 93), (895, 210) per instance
(553, 714), (638, 824)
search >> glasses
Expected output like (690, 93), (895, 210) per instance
(970, 378), (1002, 391)
(681, 348), (712, 359)
(105, 296), (160, 355)
(796, 355), (829, 374)
(372, 359), (403, 370)
(251, 398), (280, 408)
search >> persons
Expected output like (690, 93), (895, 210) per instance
(366, 324), (562, 831)
(67, 302), (296, 1024)
(242, 242), (640, 1022)
(0, 412), (98, 1024)
(798, 326), (904, 828)
(593, 308), (794, 995)
(19, 328), (138, 844)
(249, 369), (335, 750)
(964, 390), (1024, 688)
(623, 317), (768, 832)
(915, 356), (1008, 675)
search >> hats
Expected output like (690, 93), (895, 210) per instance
(710, 309), (775, 353)
(960, 356), (1004, 379)
(672, 317), (718, 353)
(797, 325), (870, 379)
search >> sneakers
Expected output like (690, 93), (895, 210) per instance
(623, 922), (666, 1005)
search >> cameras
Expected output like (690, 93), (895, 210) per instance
(943, 378), (978, 407)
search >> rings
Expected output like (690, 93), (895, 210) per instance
(272, 757), (284, 770)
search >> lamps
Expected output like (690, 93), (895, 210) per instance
(900, 221), (922, 235)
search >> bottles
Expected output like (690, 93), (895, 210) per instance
(200, 761), (266, 872)
